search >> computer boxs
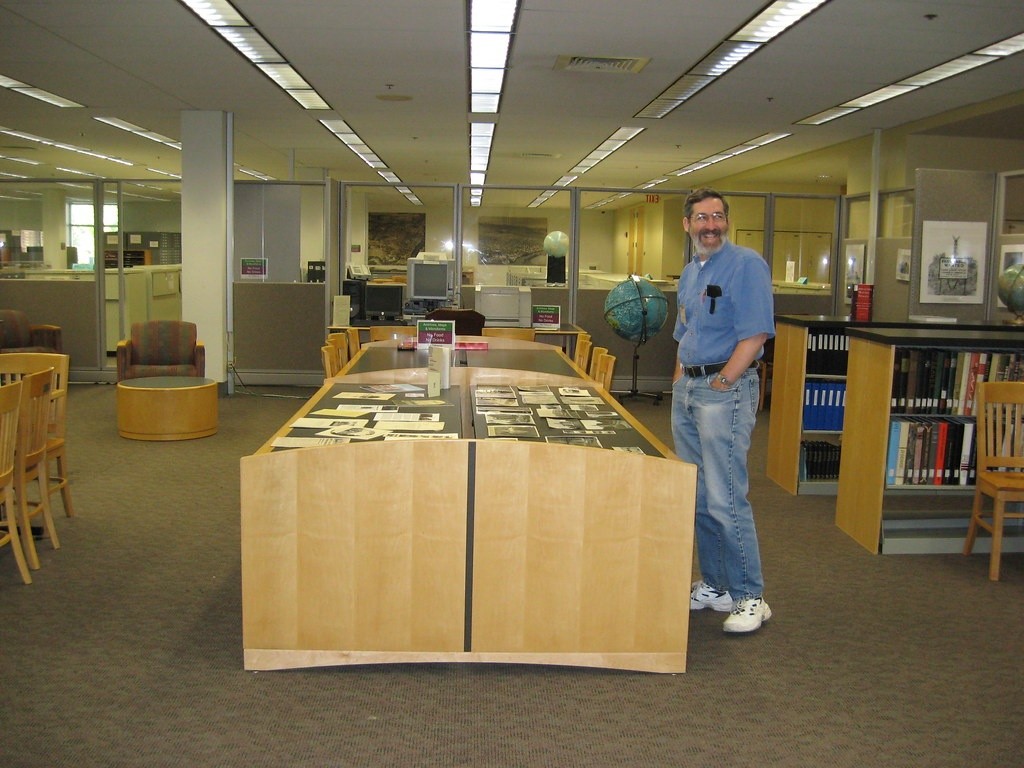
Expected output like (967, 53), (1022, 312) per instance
(343, 280), (367, 326)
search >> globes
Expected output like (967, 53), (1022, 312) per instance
(544, 231), (570, 258)
(604, 273), (669, 401)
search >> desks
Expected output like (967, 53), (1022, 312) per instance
(239, 337), (698, 675)
(117, 376), (219, 441)
(328, 324), (589, 358)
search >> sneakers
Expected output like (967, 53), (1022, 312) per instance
(722, 598), (772, 632)
(691, 582), (733, 612)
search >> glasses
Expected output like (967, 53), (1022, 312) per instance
(687, 211), (727, 223)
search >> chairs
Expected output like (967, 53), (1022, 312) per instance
(962, 381), (1024, 581)
(575, 340), (592, 374)
(596, 354), (616, 394)
(589, 347), (608, 383)
(574, 334), (591, 366)
(0, 366), (60, 571)
(347, 329), (360, 359)
(325, 339), (340, 374)
(320, 345), (336, 381)
(482, 328), (536, 342)
(0, 353), (73, 518)
(0, 382), (33, 584)
(328, 333), (348, 368)
(370, 325), (417, 342)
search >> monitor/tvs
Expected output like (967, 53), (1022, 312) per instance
(406, 252), (455, 308)
(364, 285), (403, 320)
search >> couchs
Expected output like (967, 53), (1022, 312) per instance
(116, 320), (206, 383)
(0, 310), (62, 402)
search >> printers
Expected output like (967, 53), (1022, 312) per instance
(474, 285), (532, 328)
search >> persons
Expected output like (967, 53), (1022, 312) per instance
(671, 187), (775, 633)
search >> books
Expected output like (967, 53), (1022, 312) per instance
(800, 440), (842, 481)
(804, 327), (850, 376)
(883, 416), (1024, 486)
(848, 284), (875, 323)
(891, 348), (1024, 415)
(801, 379), (846, 432)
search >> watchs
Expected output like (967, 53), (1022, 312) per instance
(717, 374), (731, 387)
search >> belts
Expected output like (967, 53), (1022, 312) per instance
(682, 360), (759, 377)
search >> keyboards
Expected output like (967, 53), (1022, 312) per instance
(352, 320), (408, 326)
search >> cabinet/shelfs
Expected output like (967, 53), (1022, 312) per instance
(765, 315), (1015, 497)
(834, 326), (1024, 555)
